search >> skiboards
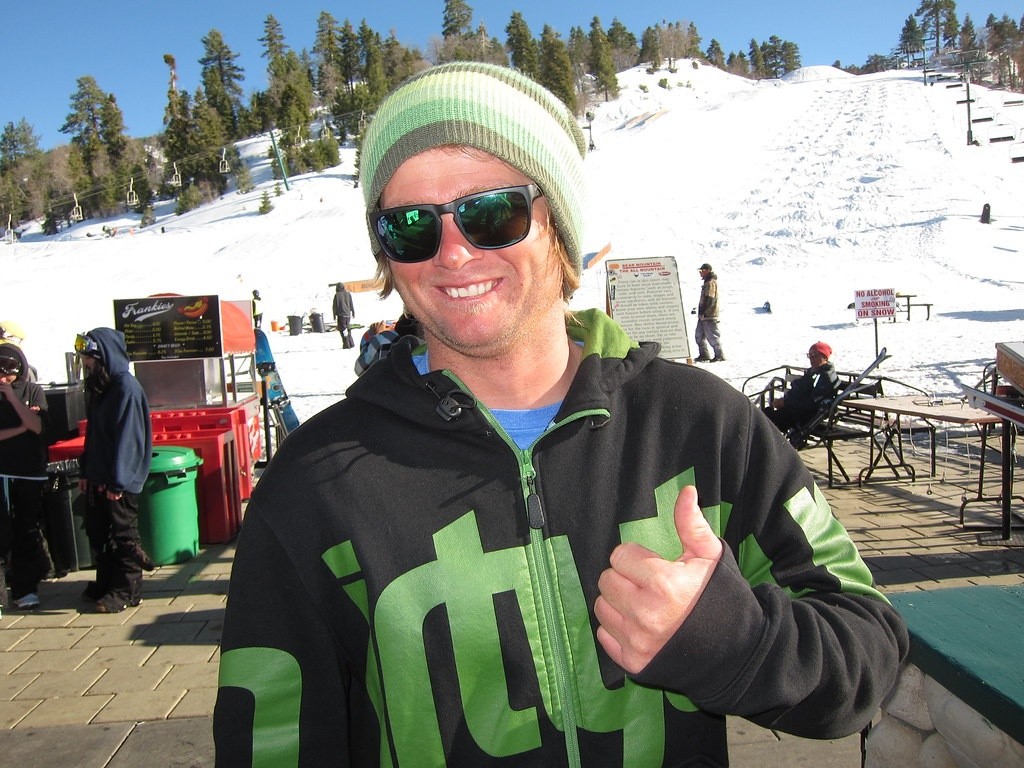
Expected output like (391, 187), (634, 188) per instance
(789, 347), (893, 446)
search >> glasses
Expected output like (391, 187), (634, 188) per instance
(806, 353), (822, 358)
(74, 334), (101, 352)
(369, 183), (544, 263)
(0, 356), (23, 373)
(698, 269), (707, 273)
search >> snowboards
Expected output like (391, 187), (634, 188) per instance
(254, 328), (300, 433)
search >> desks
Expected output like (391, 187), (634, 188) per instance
(841, 396), (1024, 523)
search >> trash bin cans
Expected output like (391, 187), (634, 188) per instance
(138, 446), (204, 566)
(287, 315), (303, 336)
(48, 470), (97, 571)
(308, 313), (325, 333)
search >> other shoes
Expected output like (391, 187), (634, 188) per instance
(89, 593), (143, 612)
(694, 356), (710, 363)
(709, 355), (725, 362)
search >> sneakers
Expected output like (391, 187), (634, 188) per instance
(14, 594), (40, 610)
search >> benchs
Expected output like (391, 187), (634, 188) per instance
(768, 375), (936, 487)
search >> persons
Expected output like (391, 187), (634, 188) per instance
(75, 327), (152, 613)
(763, 342), (841, 432)
(139, 204), (155, 228)
(353, 310), (424, 378)
(333, 282), (356, 349)
(102, 225), (115, 237)
(252, 290), (263, 328)
(0, 320), (55, 609)
(209, 63), (911, 768)
(694, 263), (724, 362)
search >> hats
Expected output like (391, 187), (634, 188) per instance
(80, 335), (104, 361)
(1, 347), (25, 375)
(810, 340), (832, 360)
(357, 60), (586, 274)
(699, 263), (714, 270)
